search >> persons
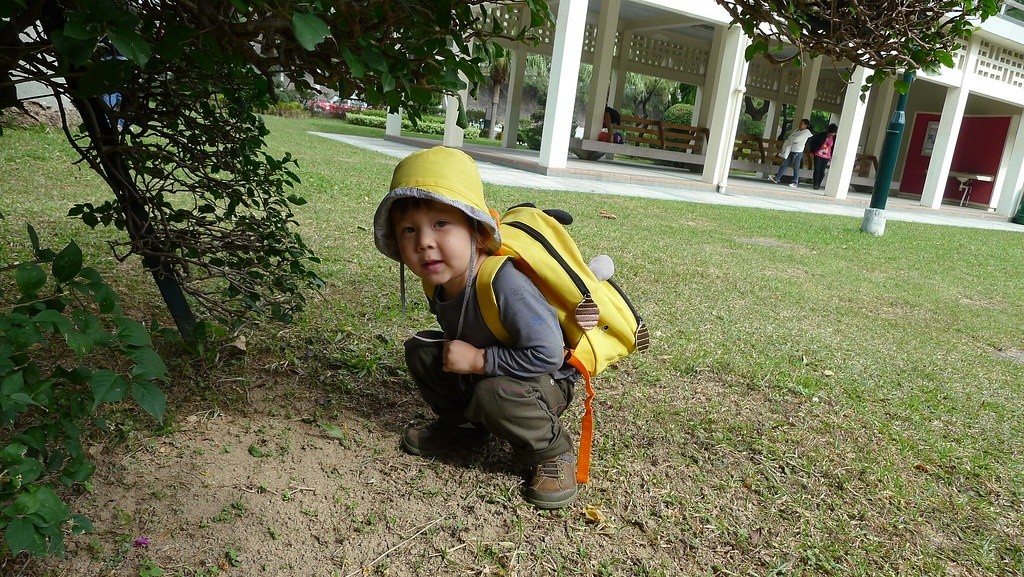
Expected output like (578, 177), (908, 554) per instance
(812, 124), (838, 190)
(374, 144), (581, 510)
(768, 119), (813, 189)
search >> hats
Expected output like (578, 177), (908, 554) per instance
(373, 145), (496, 262)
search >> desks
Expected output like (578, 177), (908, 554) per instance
(925, 170), (995, 207)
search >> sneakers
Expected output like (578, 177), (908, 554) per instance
(528, 447), (577, 508)
(403, 417), (488, 456)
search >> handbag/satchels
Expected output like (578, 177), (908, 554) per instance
(778, 140), (792, 159)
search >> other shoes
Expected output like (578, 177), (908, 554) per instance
(768, 174), (777, 184)
(789, 183), (798, 188)
(814, 184), (819, 190)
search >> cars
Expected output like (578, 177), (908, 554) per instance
(302, 93), (369, 119)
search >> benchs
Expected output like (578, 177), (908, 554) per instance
(605, 113), (710, 172)
(732, 134), (804, 184)
(805, 145), (878, 185)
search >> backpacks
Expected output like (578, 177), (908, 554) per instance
(420, 203), (649, 376)
